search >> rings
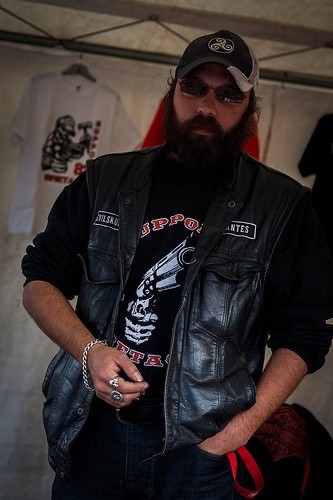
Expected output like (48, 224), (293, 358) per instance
(108, 390), (122, 404)
(108, 375), (119, 390)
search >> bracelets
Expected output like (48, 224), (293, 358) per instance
(82, 338), (108, 392)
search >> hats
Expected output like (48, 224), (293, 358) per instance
(176, 30), (260, 93)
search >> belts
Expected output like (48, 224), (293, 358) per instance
(94, 398), (164, 426)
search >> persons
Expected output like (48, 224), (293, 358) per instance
(20, 30), (333, 500)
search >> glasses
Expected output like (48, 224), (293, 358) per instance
(174, 78), (250, 104)
